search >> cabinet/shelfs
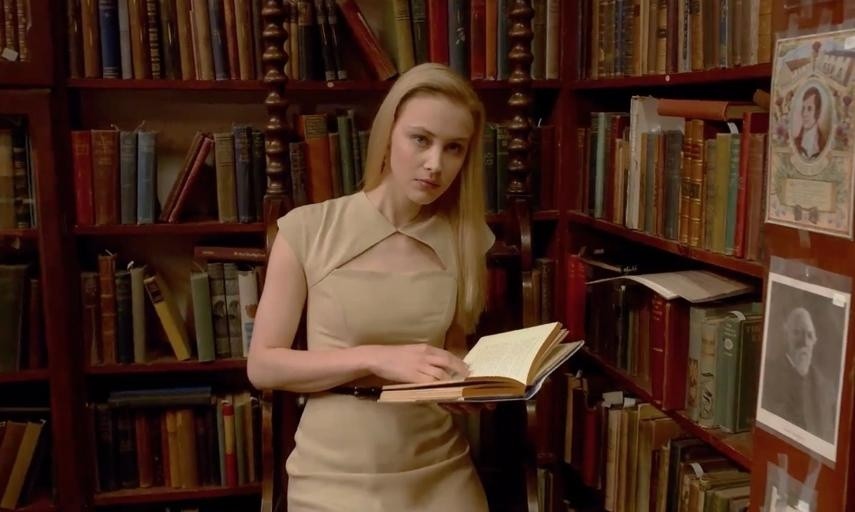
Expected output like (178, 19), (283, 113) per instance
(0, 1), (855, 511)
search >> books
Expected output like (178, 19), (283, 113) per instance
(552, 244), (759, 509)
(2, 0), (566, 86)
(375, 319), (584, 403)
(1, 246), (272, 371)
(1, 380), (259, 512)
(0, 108), (374, 229)
(576, 1), (757, 260)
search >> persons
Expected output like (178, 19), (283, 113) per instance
(243, 64), (496, 510)
(795, 86), (826, 159)
(766, 307), (834, 443)
